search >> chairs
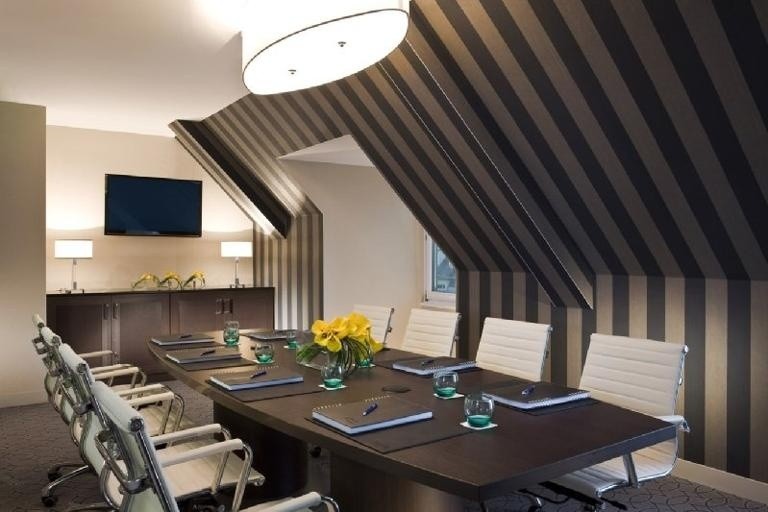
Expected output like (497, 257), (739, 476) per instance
(42, 344), (264, 504)
(475, 316), (554, 382)
(91, 382), (338, 512)
(352, 302), (394, 347)
(40, 327), (164, 425)
(401, 309), (462, 358)
(520, 333), (690, 509)
(32, 313), (155, 412)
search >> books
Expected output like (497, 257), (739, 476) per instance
(152, 333), (214, 346)
(209, 366), (304, 392)
(392, 356), (477, 375)
(165, 347), (243, 364)
(481, 382), (591, 410)
(312, 395), (433, 435)
(252, 333), (297, 339)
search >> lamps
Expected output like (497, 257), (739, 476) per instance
(241, 0), (410, 94)
(54, 238), (93, 293)
(220, 242), (254, 288)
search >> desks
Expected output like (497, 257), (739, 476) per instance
(146, 327), (676, 511)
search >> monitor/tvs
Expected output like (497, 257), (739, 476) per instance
(103, 173), (203, 238)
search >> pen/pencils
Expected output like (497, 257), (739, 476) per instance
(250, 371), (266, 378)
(179, 334), (192, 338)
(200, 350), (215, 356)
(363, 403), (377, 416)
(422, 359), (434, 365)
(522, 385), (536, 395)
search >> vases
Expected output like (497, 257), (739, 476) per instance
(324, 351), (342, 365)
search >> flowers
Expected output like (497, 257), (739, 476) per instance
(296, 311), (385, 377)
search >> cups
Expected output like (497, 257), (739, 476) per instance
(287, 331), (297, 349)
(354, 346), (374, 368)
(223, 321), (239, 345)
(432, 370), (459, 397)
(464, 393), (494, 427)
(255, 343), (275, 363)
(321, 362), (344, 388)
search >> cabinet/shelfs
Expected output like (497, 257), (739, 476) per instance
(172, 289), (273, 336)
(49, 294), (169, 376)
